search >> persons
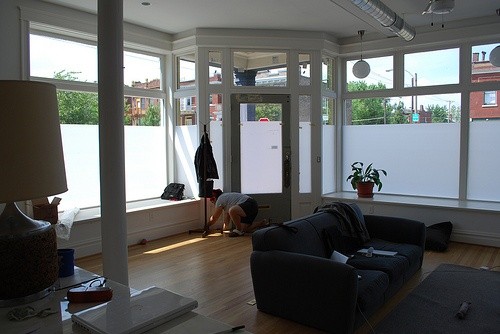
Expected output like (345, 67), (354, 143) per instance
(206, 188), (268, 238)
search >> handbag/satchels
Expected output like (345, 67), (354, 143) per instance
(161, 183), (184, 200)
(198, 178), (214, 198)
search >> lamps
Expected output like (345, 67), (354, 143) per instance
(0, 78), (70, 308)
(352, 30), (370, 79)
(490, 8), (500, 66)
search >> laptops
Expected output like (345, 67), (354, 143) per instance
(72, 286), (198, 334)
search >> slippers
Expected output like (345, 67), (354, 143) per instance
(263, 217), (271, 228)
(228, 229), (245, 237)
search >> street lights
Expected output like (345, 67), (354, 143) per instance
(386, 69), (414, 113)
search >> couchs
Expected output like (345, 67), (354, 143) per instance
(251, 202), (425, 333)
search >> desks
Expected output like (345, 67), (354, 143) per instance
(0, 266), (257, 334)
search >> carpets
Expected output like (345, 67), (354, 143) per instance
(367, 263), (500, 334)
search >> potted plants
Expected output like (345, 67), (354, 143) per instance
(346, 161), (387, 198)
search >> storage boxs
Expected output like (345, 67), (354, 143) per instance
(25, 195), (62, 225)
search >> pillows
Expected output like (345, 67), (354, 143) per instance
(425, 220), (453, 252)
